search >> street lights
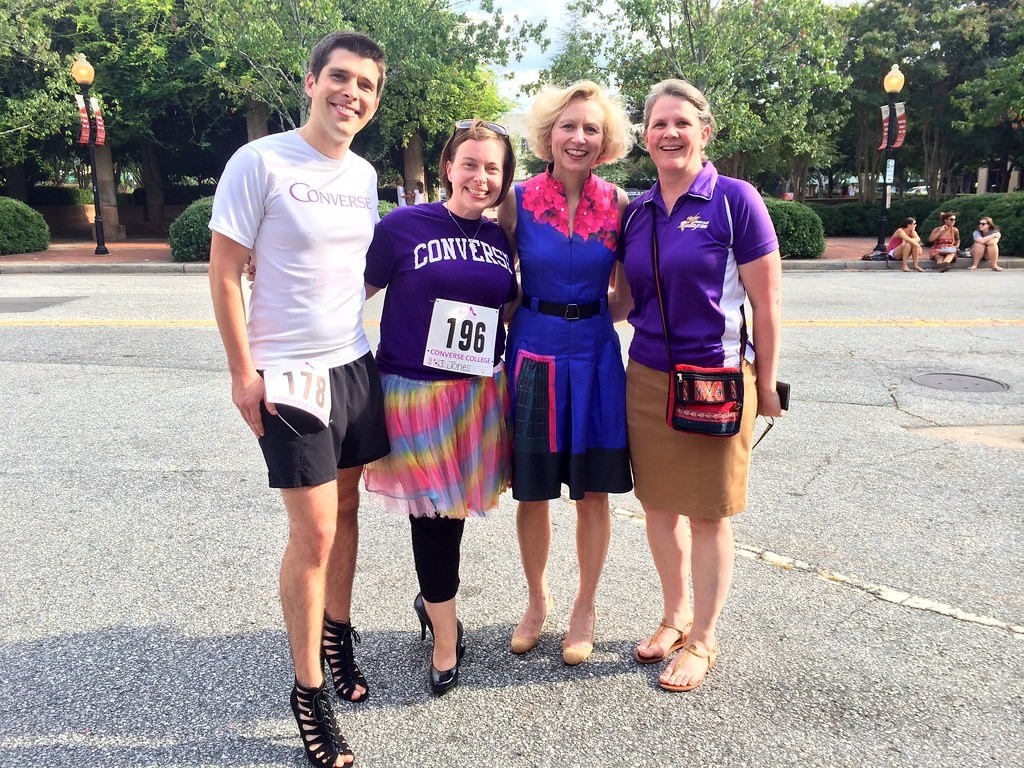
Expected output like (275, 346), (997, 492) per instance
(71, 52), (109, 255)
(871, 63), (905, 252)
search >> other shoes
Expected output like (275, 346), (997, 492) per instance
(968, 266), (977, 270)
(562, 603), (597, 664)
(511, 591), (554, 653)
(913, 266), (925, 272)
(902, 266), (911, 272)
(992, 265), (1003, 272)
(937, 262), (952, 273)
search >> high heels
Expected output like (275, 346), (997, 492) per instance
(414, 592), (465, 658)
(319, 617), (369, 703)
(429, 619), (462, 694)
(289, 670), (355, 768)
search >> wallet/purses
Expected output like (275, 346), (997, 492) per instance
(777, 382), (789, 410)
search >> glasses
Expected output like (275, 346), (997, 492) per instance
(453, 119), (509, 146)
(947, 218), (957, 222)
(911, 222), (917, 226)
(751, 411), (775, 451)
(978, 223), (989, 226)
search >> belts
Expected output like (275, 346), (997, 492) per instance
(522, 295), (609, 321)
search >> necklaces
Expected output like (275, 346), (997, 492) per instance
(447, 201), (483, 250)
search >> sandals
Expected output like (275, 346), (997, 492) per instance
(635, 613), (696, 663)
(658, 637), (718, 691)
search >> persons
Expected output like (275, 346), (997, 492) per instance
(969, 217), (1003, 271)
(497, 79), (635, 665)
(886, 217), (925, 271)
(928, 212), (961, 273)
(841, 182), (856, 197)
(207, 30), (391, 768)
(245, 117), (523, 694)
(610, 79), (785, 692)
(408, 182), (425, 204)
(395, 176), (410, 207)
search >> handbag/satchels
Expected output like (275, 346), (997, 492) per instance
(666, 364), (745, 437)
